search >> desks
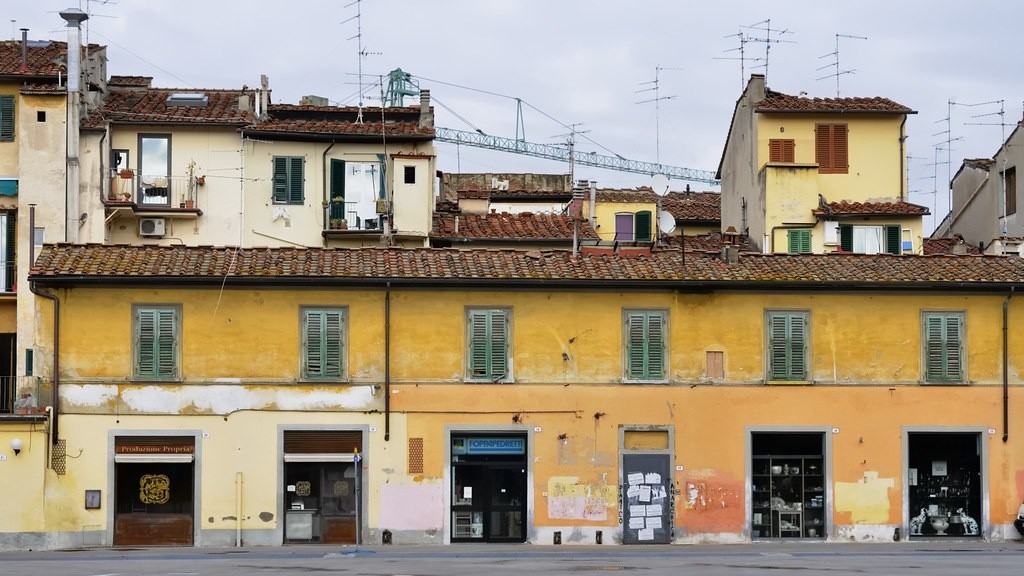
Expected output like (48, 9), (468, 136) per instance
(285, 510), (313, 543)
(779, 511), (802, 538)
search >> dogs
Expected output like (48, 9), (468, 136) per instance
(909, 507), (929, 536)
(956, 506), (980, 535)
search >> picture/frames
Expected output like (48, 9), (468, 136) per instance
(931, 460), (948, 476)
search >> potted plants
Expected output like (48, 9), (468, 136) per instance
(331, 196), (344, 210)
(120, 168), (134, 178)
(331, 218), (340, 228)
(180, 193), (185, 208)
(340, 219), (348, 228)
(184, 157), (194, 209)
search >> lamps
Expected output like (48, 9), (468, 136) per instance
(10, 438), (22, 456)
(370, 384), (380, 396)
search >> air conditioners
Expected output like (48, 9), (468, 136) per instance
(139, 217), (166, 236)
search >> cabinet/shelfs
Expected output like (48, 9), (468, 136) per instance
(752, 454), (824, 538)
